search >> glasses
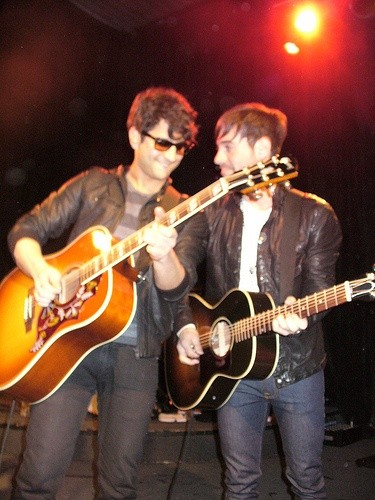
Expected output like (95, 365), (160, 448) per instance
(141, 130), (189, 154)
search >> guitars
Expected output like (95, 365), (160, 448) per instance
(166, 265), (374, 411)
(0, 153), (299, 406)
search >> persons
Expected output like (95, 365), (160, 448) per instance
(170, 103), (344, 500)
(7, 86), (205, 500)
